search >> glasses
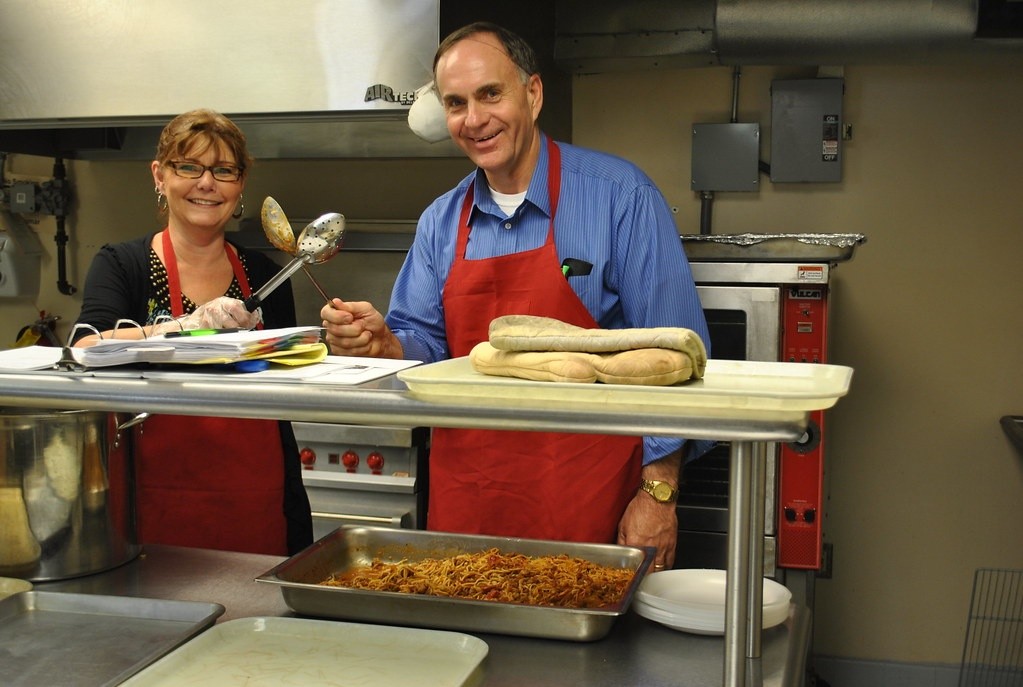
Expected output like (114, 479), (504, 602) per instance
(168, 160), (243, 182)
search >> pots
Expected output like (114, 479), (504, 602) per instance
(0, 409), (150, 582)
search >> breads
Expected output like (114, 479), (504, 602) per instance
(470, 341), (597, 383)
(563, 348), (693, 386)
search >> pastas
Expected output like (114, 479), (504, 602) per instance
(314, 547), (638, 613)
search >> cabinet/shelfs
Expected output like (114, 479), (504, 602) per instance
(0, 346), (812, 687)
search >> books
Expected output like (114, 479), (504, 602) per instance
(0, 323), (328, 373)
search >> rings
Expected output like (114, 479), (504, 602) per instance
(654, 565), (665, 568)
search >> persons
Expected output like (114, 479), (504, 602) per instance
(319, 22), (711, 577)
(70, 108), (314, 556)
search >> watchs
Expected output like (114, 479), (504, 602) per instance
(640, 476), (679, 504)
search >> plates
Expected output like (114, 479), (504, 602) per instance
(632, 568), (792, 636)
